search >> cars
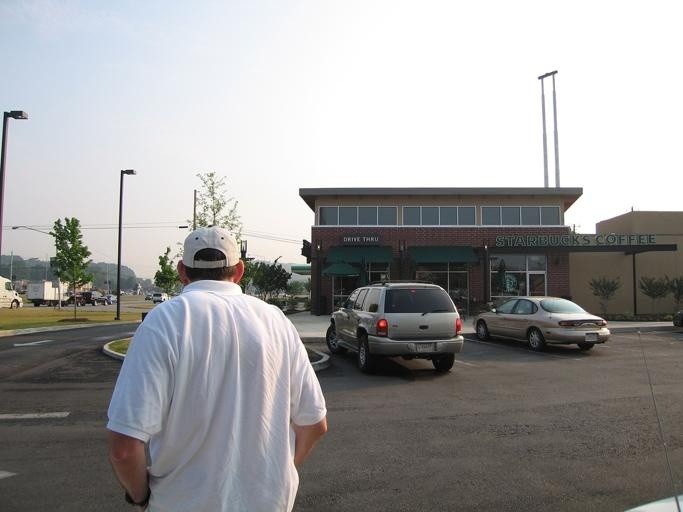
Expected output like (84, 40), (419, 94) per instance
(472, 295), (611, 352)
(70, 290), (117, 307)
(145, 292), (168, 304)
(326, 279), (464, 375)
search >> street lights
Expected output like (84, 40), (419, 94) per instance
(114, 169), (136, 319)
(0, 110), (28, 259)
(12, 225), (61, 311)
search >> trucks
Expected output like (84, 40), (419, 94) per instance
(27, 280), (70, 307)
(0, 275), (23, 309)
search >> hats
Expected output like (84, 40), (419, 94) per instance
(181, 226), (240, 269)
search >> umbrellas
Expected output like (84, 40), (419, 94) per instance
(322, 261), (359, 304)
(493, 258), (509, 296)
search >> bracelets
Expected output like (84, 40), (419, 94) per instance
(124, 485), (151, 508)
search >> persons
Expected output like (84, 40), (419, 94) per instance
(105, 224), (327, 512)
